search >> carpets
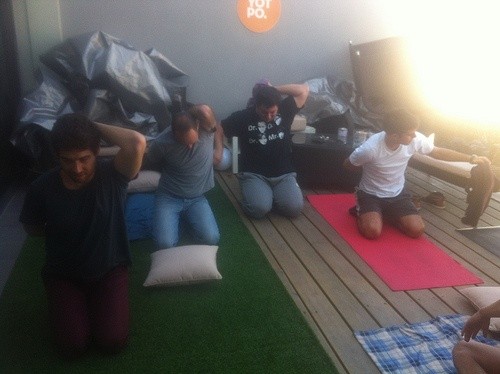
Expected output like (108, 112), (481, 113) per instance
(0, 175), (339, 374)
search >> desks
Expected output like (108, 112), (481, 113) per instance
(408, 157), (498, 227)
(293, 133), (363, 192)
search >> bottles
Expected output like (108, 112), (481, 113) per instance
(338, 127), (347, 145)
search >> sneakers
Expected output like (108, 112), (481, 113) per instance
(403, 193), (421, 211)
(422, 190), (446, 210)
